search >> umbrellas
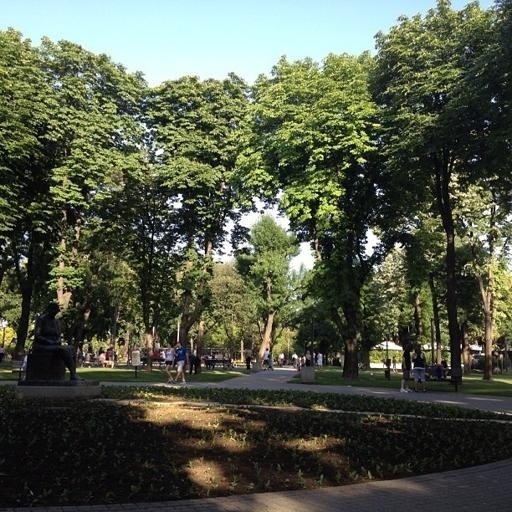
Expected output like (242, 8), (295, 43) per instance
(372, 340), (403, 358)
(422, 342), (443, 351)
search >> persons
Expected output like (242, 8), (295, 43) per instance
(141, 347), (160, 366)
(165, 342), (187, 383)
(81, 344), (113, 367)
(391, 343), (482, 393)
(204, 354), (226, 368)
(245, 346), (252, 369)
(33, 302), (86, 382)
(263, 348), (322, 371)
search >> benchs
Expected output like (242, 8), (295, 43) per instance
(411, 366), (459, 392)
(205, 358), (231, 370)
(140, 358), (165, 367)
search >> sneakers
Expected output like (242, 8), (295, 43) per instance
(406, 388), (413, 392)
(400, 389), (408, 393)
